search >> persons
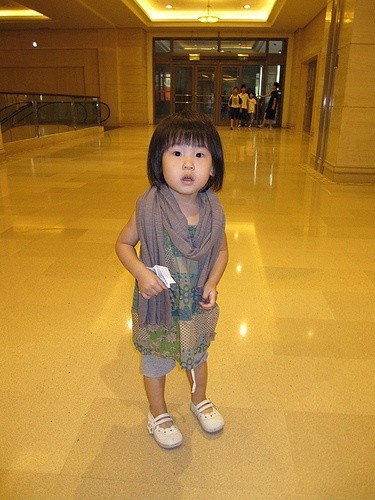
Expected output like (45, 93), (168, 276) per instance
(115, 109), (229, 449)
(235, 84), (257, 130)
(257, 82), (279, 128)
(228, 86), (243, 129)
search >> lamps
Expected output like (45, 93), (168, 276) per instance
(196, 0), (222, 24)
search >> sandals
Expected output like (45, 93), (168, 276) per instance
(146, 408), (183, 449)
(190, 398), (224, 432)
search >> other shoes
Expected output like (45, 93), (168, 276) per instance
(238, 124), (241, 128)
(248, 124), (252, 128)
(230, 126), (233, 130)
(258, 124), (264, 128)
